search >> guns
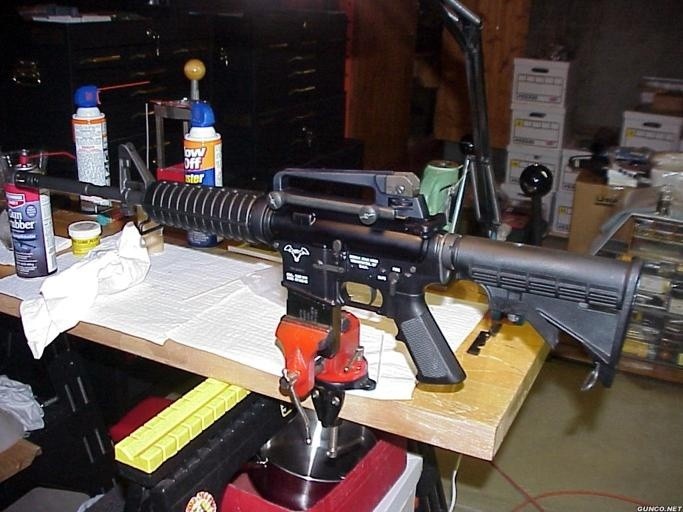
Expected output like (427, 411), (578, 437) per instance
(14, 142), (644, 391)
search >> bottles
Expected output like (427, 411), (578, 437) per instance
(183, 101), (224, 250)
(68, 221), (102, 257)
(71, 105), (112, 213)
(3, 148), (59, 279)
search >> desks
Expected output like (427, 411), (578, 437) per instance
(0, 208), (551, 511)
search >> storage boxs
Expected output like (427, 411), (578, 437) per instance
(499, 55), (683, 253)
